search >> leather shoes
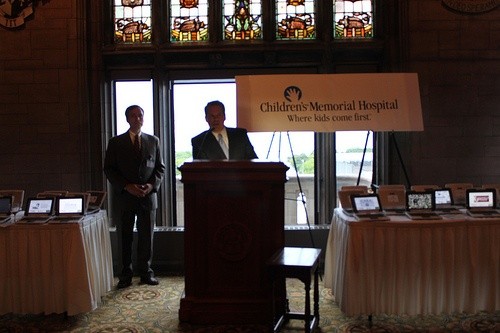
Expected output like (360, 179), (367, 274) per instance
(116, 280), (131, 288)
(140, 277), (158, 285)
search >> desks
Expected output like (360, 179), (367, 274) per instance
(265, 247), (322, 333)
(0, 210), (115, 317)
(323, 205), (500, 316)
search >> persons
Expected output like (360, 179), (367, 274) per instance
(104, 105), (166, 289)
(191, 101), (258, 159)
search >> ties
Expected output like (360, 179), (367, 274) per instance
(134, 134), (139, 154)
(217, 133), (228, 160)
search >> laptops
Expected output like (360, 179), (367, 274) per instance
(0, 190), (107, 223)
(338, 184), (500, 222)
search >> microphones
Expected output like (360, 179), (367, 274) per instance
(195, 127), (214, 159)
